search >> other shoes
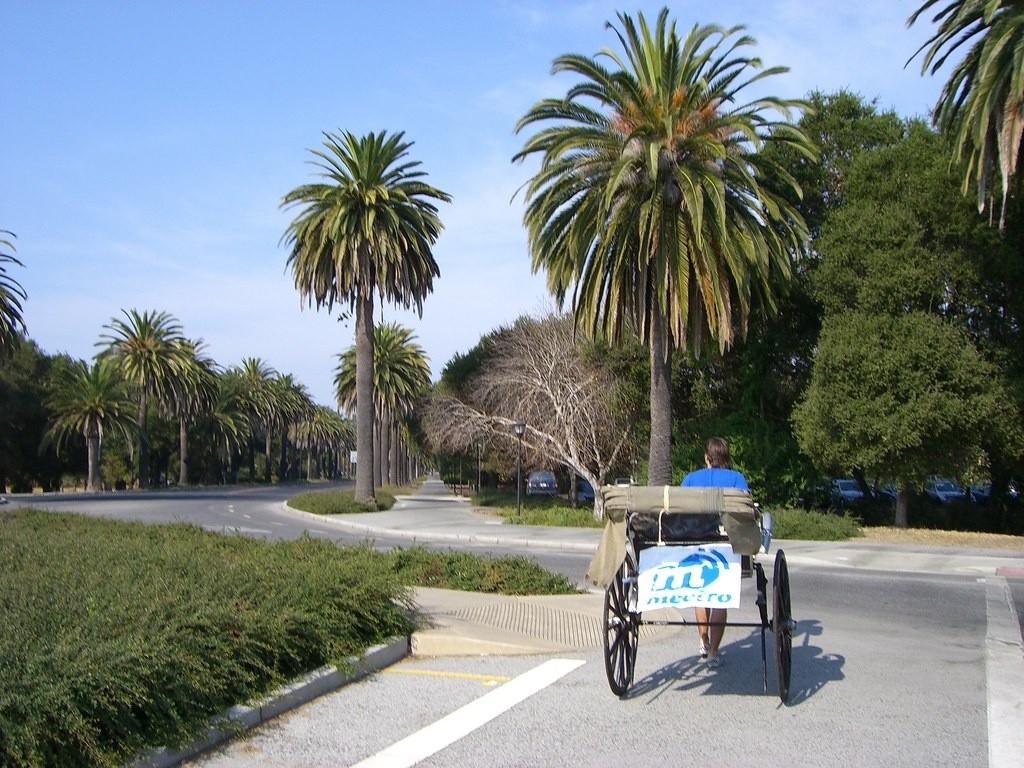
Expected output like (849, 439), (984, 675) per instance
(707, 654), (724, 669)
(700, 644), (710, 658)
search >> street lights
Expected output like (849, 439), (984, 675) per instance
(512, 419), (527, 525)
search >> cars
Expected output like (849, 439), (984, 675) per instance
(824, 479), (876, 512)
(567, 479), (595, 503)
(870, 474), (1020, 506)
(525, 469), (559, 498)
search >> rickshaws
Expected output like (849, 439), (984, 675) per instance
(601, 485), (799, 705)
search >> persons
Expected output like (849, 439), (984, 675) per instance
(679, 436), (749, 667)
